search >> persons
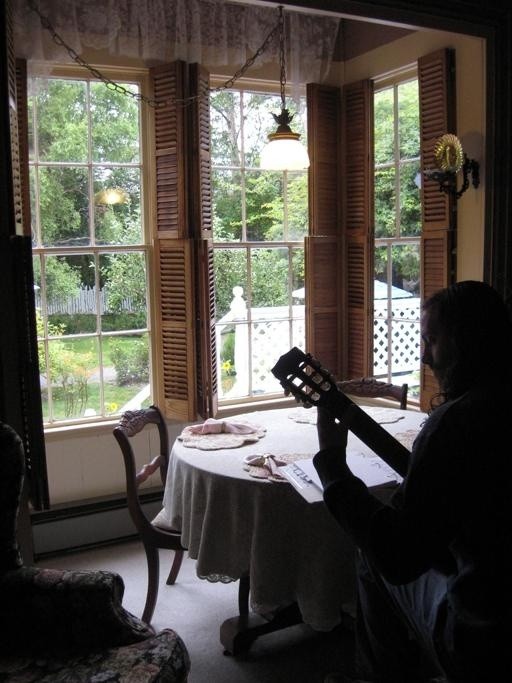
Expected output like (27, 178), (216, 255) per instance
(312, 280), (512, 682)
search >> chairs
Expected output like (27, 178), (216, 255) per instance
(0, 420), (190, 682)
(111, 403), (251, 626)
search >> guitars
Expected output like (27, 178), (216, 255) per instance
(271, 348), (412, 478)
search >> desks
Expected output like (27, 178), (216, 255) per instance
(167, 403), (429, 658)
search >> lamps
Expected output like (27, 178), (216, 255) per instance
(259, 3), (311, 171)
